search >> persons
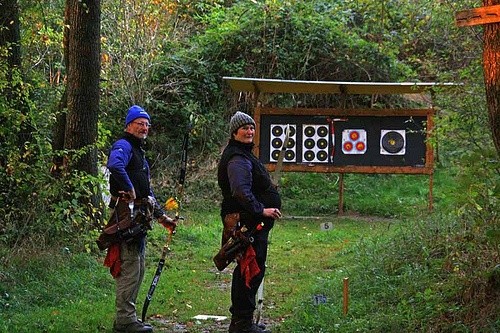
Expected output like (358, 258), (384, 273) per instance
(217, 110), (282, 333)
(106, 105), (176, 333)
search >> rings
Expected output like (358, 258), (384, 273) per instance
(274, 208), (278, 212)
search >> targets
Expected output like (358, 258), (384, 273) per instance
(379, 129), (407, 155)
(301, 123), (329, 163)
(269, 123), (297, 163)
(341, 128), (367, 155)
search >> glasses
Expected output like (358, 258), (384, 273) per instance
(133, 121), (151, 128)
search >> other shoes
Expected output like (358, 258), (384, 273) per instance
(114, 320), (152, 333)
(229, 321), (272, 333)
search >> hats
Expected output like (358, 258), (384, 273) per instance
(230, 111), (255, 134)
(126, 105), (150, 125)
